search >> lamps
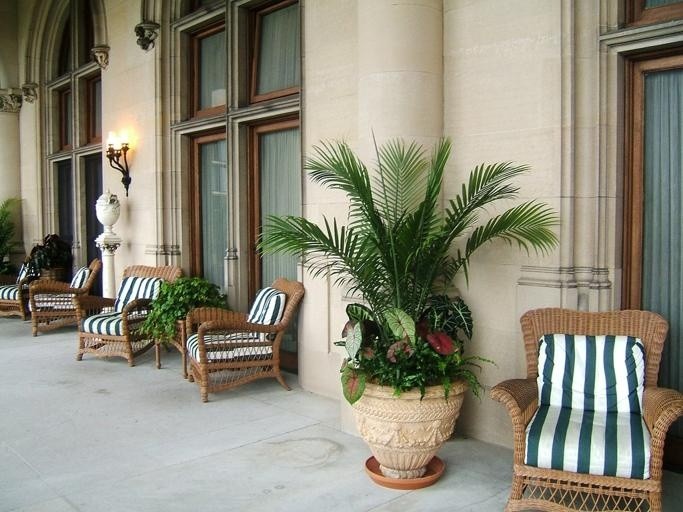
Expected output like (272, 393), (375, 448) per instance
(104, 126), (134, 198)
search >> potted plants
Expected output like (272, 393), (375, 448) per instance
(252, 125), (563, 497)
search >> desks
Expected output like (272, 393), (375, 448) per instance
(37, 269), (64, 280)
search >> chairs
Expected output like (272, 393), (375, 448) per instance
(181, 273), (306, 405)
(485, 302), (681, 511)
(0, 254), (40, 323)
(29, 255), (108, 341)
(71, 263), (184, 369)
